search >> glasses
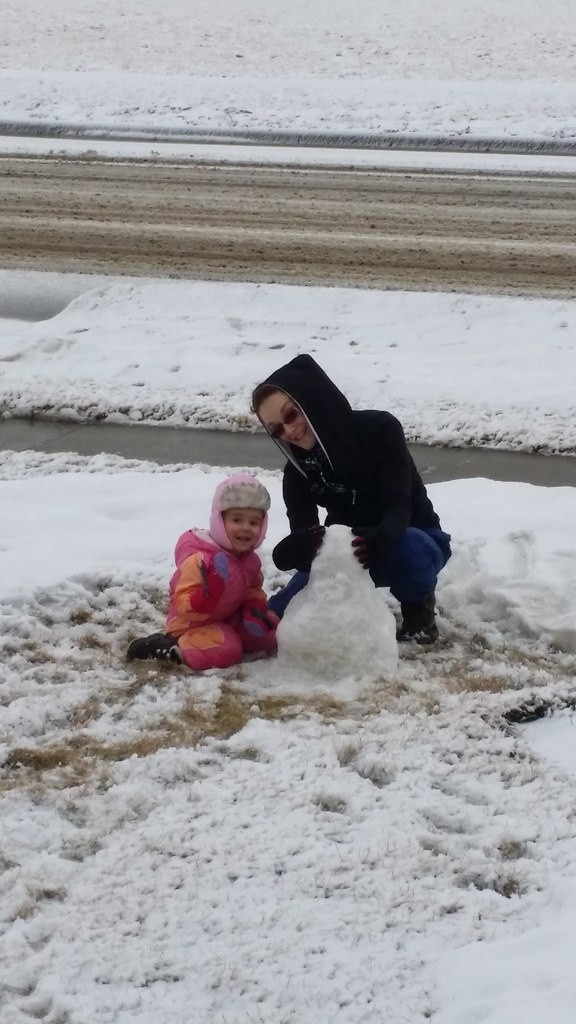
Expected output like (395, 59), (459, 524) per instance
(269, 403), (299, 439)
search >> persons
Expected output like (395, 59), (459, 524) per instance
(125, 475), (280, 671)
(249, 354), (452, 646)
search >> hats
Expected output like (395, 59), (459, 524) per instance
(209, 476), (270, 550)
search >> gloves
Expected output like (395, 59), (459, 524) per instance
(189, 552), (229, 614)
(294, 525), (326, 553)
(241, 603), (271, 653)
(351, 527), (377, 569)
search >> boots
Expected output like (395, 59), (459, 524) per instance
(127, 632), (177, 660)
(396, 592), (439, 644)
